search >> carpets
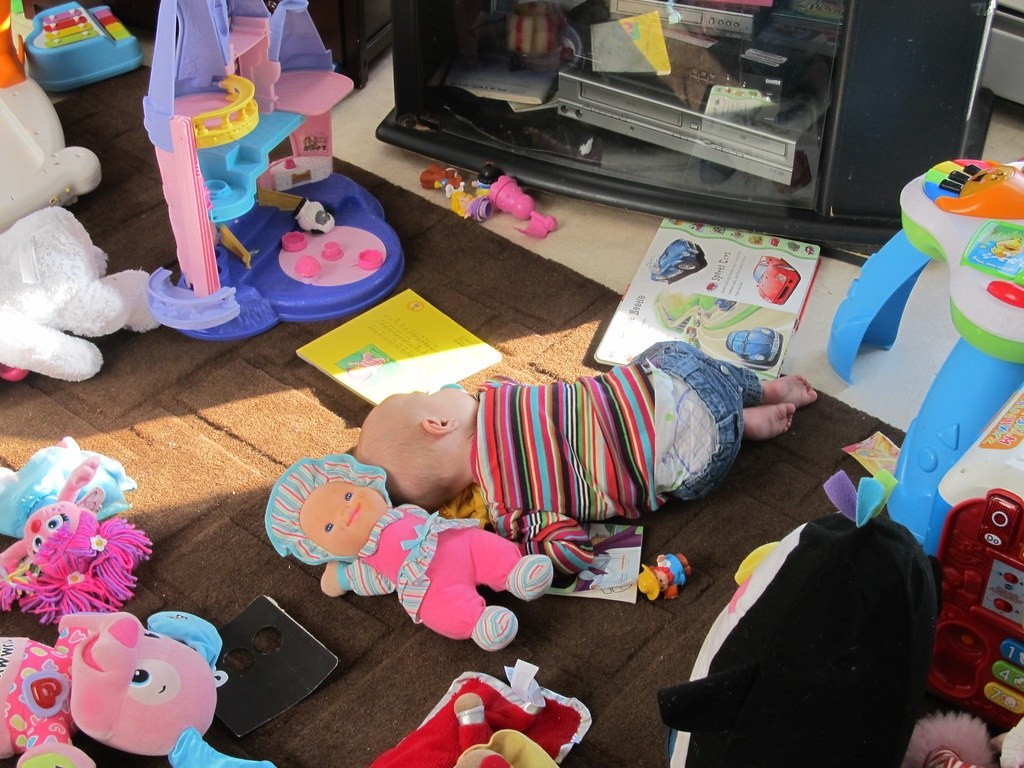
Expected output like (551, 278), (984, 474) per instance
(0, 65), (909, 768)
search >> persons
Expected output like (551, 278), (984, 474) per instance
(355, 341), (817, 578)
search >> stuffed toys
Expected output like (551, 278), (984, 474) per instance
(0, 611), (276, 768)
(0, 436), (138, 539)
(266, 453), (554, 651)
(0, 456), (154, 625)
(1, 206), (164, 381)
(657, 467), (937, 768)
(368, 659), (592, 768)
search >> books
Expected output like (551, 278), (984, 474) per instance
(545, 523), (643, 604)
(506, 91), (559, 112)
(594, 218), (822, 382)
(296, 288), (502, 408)
(443, 61), (557, 105)
